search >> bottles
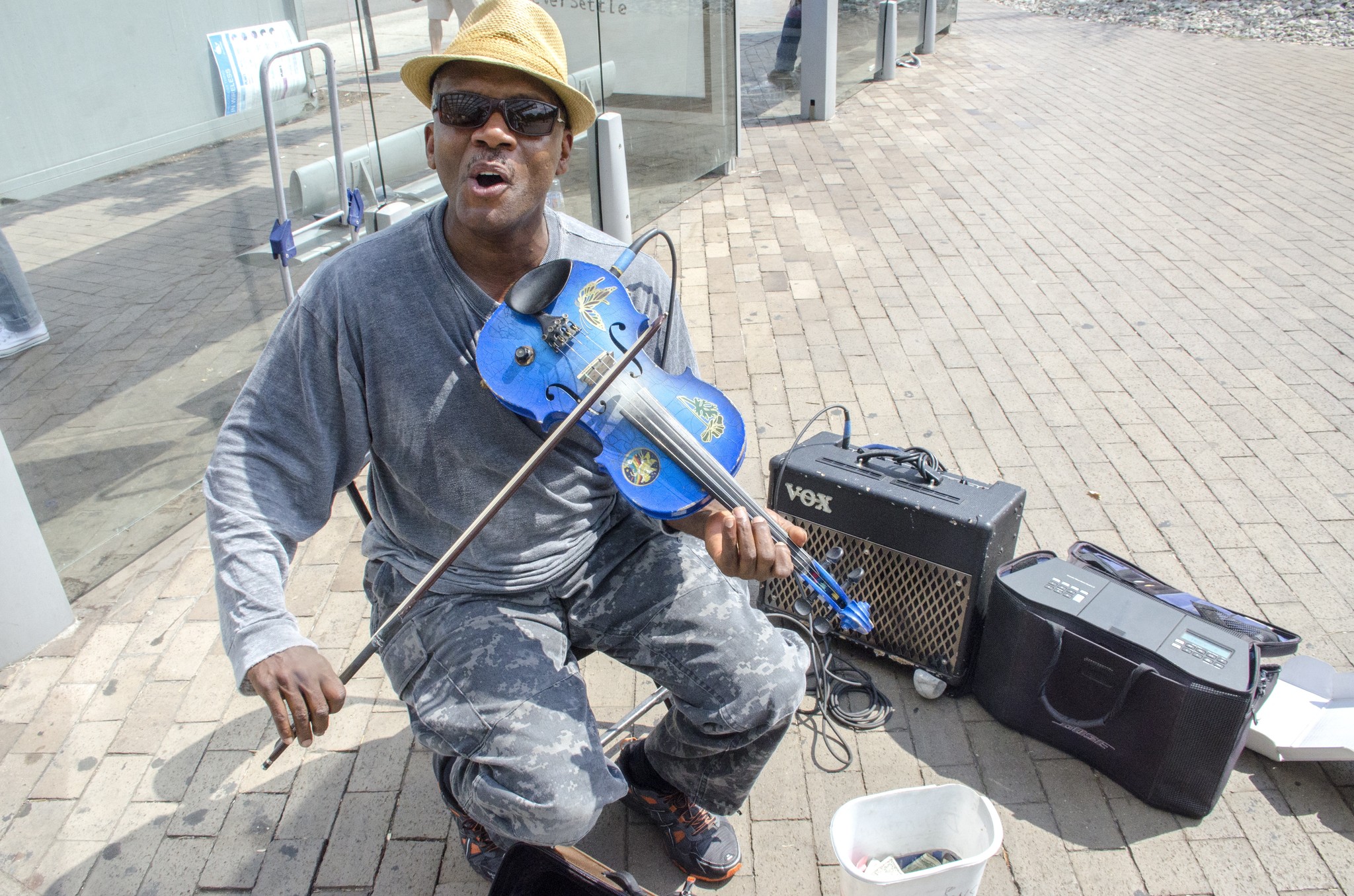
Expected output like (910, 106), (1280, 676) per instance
(544, 177), (566, 213)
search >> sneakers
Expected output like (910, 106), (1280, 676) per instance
(614, 737), (742, 882)
(432, 752), (508, 883)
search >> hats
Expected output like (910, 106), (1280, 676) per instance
(400, 0), (596, 136)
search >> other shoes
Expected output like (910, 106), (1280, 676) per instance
(795, 63), (801, 72)
(767, 69), (790, 79)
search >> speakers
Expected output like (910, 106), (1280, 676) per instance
(757, 432), (1027, 695)
(995, 556), (1257, 690)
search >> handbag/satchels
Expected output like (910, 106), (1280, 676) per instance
(971, 540), (1302, 820)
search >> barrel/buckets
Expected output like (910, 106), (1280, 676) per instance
(830, 782), (1004, 896)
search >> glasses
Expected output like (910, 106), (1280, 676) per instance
(430, 91), (566, 136)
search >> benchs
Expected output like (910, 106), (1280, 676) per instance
(234, 60), (615, 264)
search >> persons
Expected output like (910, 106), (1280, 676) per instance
(204, 0), (810, 880)
(767, 0), (805, 90)
(414, 0), (482, 58)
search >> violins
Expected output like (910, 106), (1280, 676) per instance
(471, 258), (881, 639)
